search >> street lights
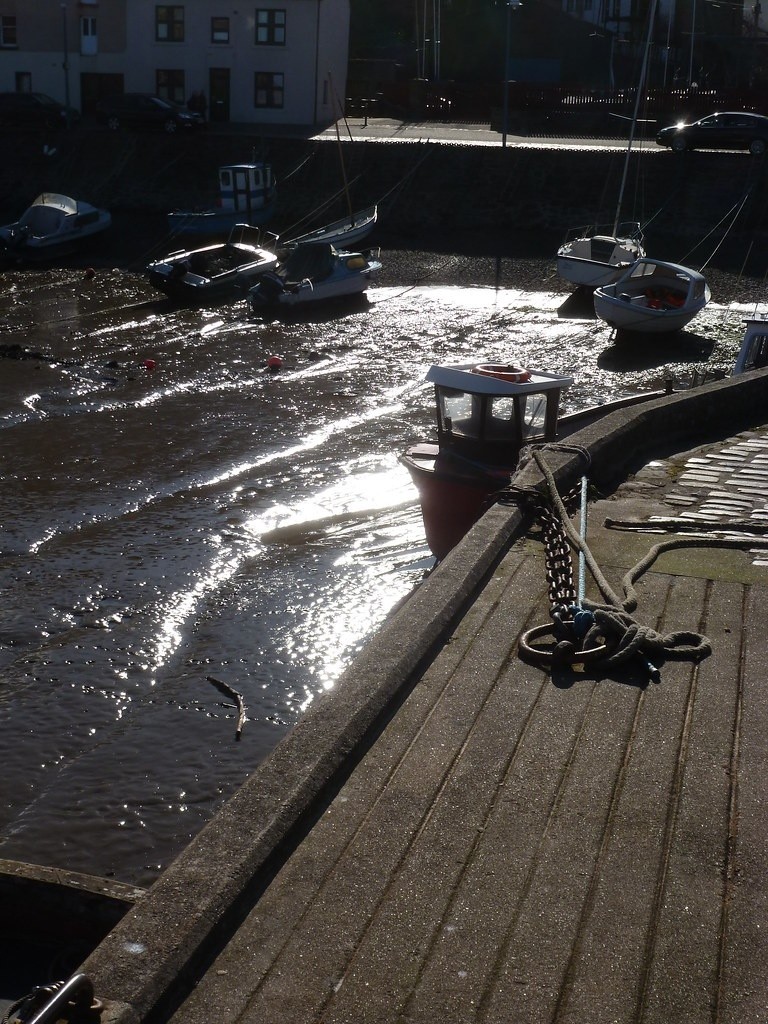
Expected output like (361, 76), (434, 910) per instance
(502, 0), (523, 146)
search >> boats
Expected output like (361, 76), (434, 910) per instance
(0, 192), (112, 260)
(245, 243), (382, 307)
(166, 148), (278, 241)
(398, 319), (768, 562)
(145, 224), (279, 295)
(593, 258), (712, 333)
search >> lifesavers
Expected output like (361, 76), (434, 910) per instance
(473, 364), (530, 382)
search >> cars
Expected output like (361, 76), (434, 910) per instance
(85, 93), (206, 135)
(0, 92), (81, 130)
(656, 112), (768, 155)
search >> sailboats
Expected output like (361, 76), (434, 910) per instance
(556, 0), (657, 286)
(265, 71), (377, 250)
